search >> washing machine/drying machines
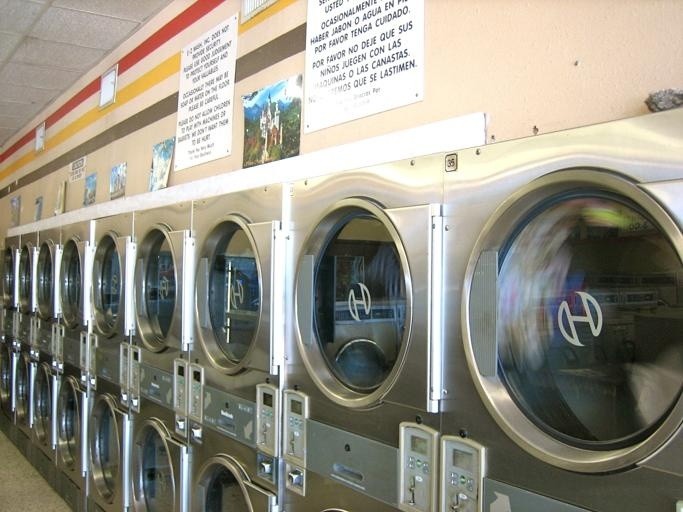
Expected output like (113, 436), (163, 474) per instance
(0, 101), (683, 512)
